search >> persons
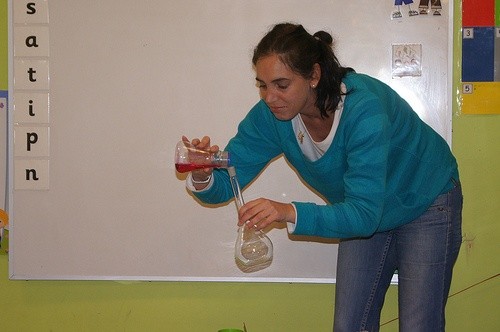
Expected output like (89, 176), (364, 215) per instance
(181, 23), (463, 331)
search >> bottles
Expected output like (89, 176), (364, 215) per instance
(175, 140), (231, 173)
(226, 166), (273, 266)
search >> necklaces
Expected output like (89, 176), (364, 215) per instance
(296, 107), (319, 144)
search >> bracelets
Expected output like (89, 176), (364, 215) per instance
(192, 175), (211, 184)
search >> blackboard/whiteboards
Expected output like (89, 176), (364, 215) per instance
(6, 0), (453, 286)
